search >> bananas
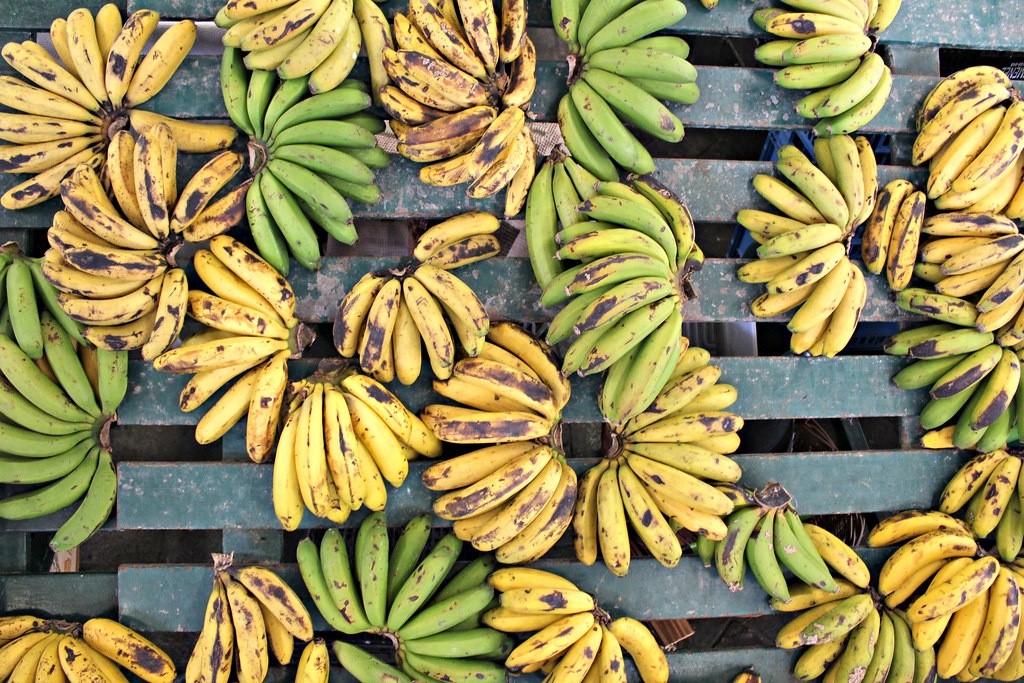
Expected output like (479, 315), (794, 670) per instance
(0, 0), (1024, 683)
